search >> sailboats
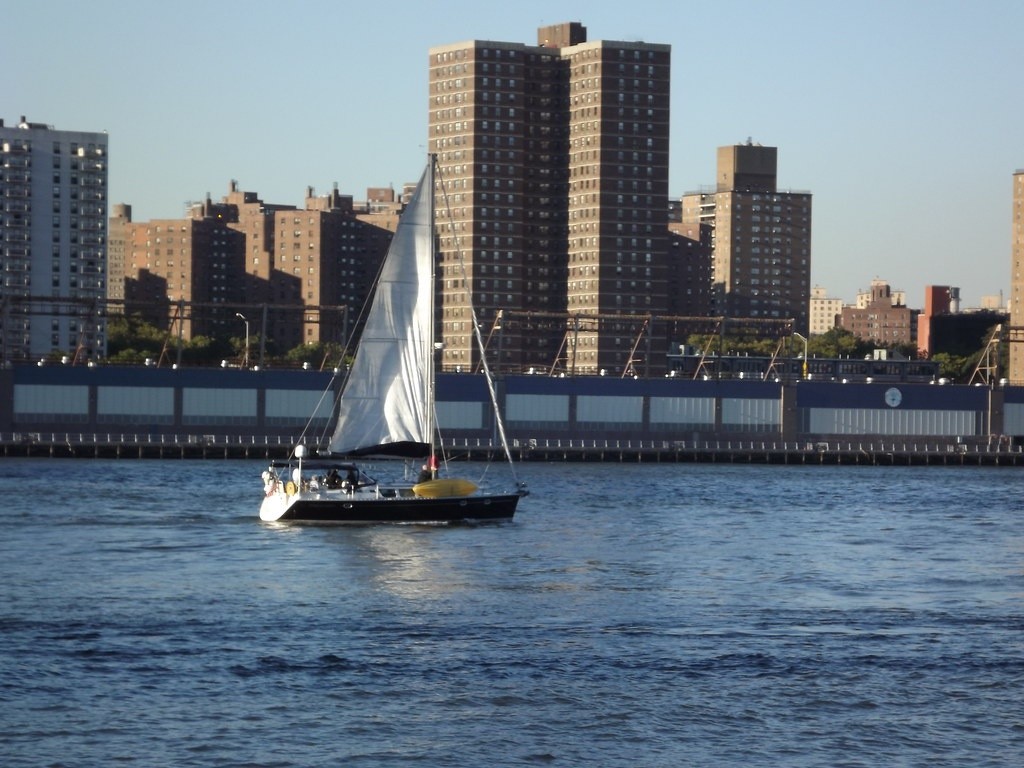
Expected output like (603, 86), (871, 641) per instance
(258, 150), (529, 523)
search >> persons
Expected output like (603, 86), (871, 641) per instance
(310, 462), (359, 490)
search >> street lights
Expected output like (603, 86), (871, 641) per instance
(235, 312), (248, 369)
